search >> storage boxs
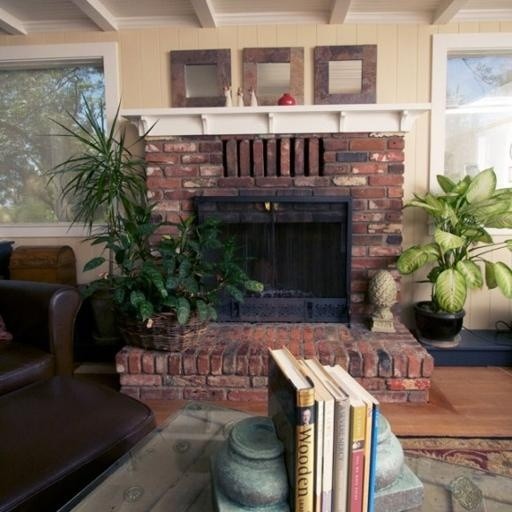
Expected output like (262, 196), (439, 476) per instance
(9, 245), (77, 286)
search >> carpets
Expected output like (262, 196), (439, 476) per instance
(396, 437), (512, 478)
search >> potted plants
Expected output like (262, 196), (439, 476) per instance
(81, 201), (264, 353)
(37, 90), (172, 353)
(397, 166), (512, 348)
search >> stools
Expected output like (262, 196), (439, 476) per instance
(0, 373), (156, 512)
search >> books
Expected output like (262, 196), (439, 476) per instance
(266, 343), (380, 511)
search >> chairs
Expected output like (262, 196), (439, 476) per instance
(1, 280), (83, 396)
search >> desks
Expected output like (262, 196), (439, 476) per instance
(56, 398), (512, 512)
